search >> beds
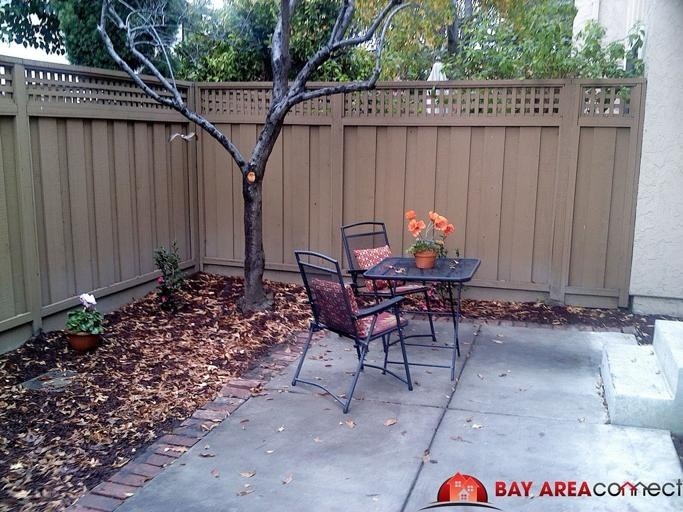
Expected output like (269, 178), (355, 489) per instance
(363, 256), (481, 380)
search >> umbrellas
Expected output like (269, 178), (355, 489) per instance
(421, 55), (450, 115)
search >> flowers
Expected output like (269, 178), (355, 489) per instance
(405, 210), (455, 257)
(64, 293), (106, 335)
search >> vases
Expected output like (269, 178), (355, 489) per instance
(65, 329), (98, 351)
(414, 250), (437, 269)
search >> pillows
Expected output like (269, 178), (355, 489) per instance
(311, 278), (367, 336)
(352, 244), (403, 292)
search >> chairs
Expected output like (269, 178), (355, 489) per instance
(340, 221), (436, 352)
(291, 250), (413, 413)
